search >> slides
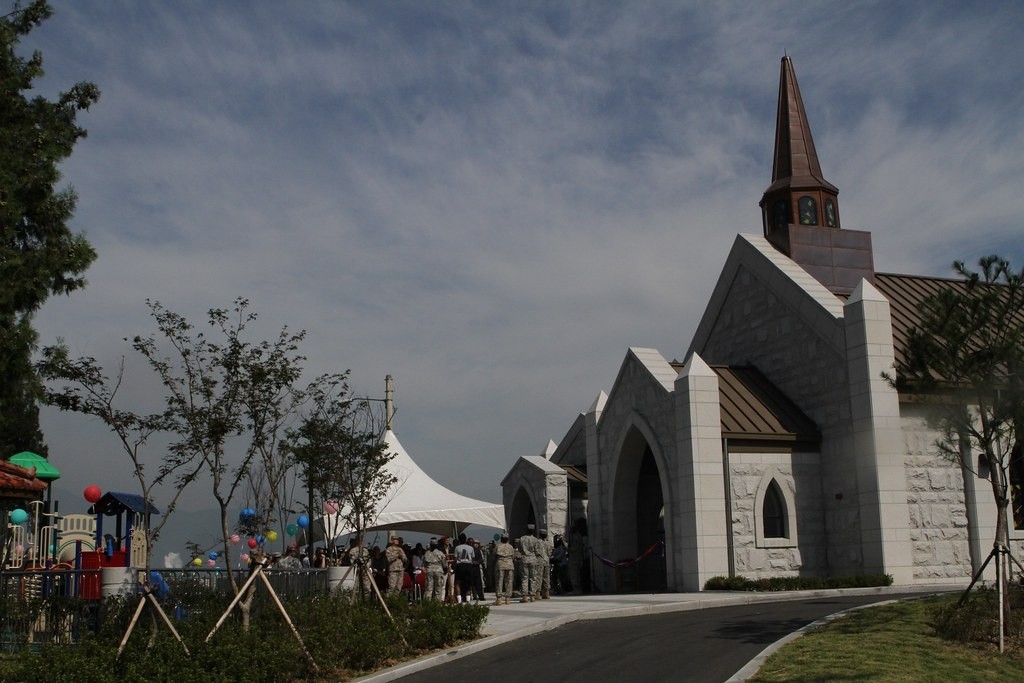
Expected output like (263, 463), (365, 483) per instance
(136, 572), (186, 626)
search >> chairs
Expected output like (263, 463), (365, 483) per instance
(414, 571), (426, 600)
(401, 572), (414, 601)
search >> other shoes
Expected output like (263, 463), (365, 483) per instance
(480, 598), (487, 601)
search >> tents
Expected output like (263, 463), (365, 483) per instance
(293, 428), (510, 556)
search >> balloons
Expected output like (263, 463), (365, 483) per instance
(84, 484), (101, 503)
(494, 533), (500, 541)
(10, 508), (27, 525)
(49, 545), (59, 553)
(15, 545), (24, 555)
(193, 498), (339, 575)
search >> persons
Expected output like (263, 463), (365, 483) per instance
(241, 523), (572, 613)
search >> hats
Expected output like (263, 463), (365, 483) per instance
(527, 524), (536, 530)
(287, 545), (297, 552)
(430, 539), (438, 545)
(474, 539), (480, 544)
(501, 533), (509, 538)
(272, 552), (282, 558)
(515, 538), (520, 542)
(539, 529), (548, 535)
(391, 536), (399, 540)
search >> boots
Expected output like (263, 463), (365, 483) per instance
(542, 591), (550, 599)
(493, 597), (502, 605)
(535, 591), (541, 599)
(505, 598), (510, 605)
(519, 596), (528, 603)
(531, 595), (536, 602)
